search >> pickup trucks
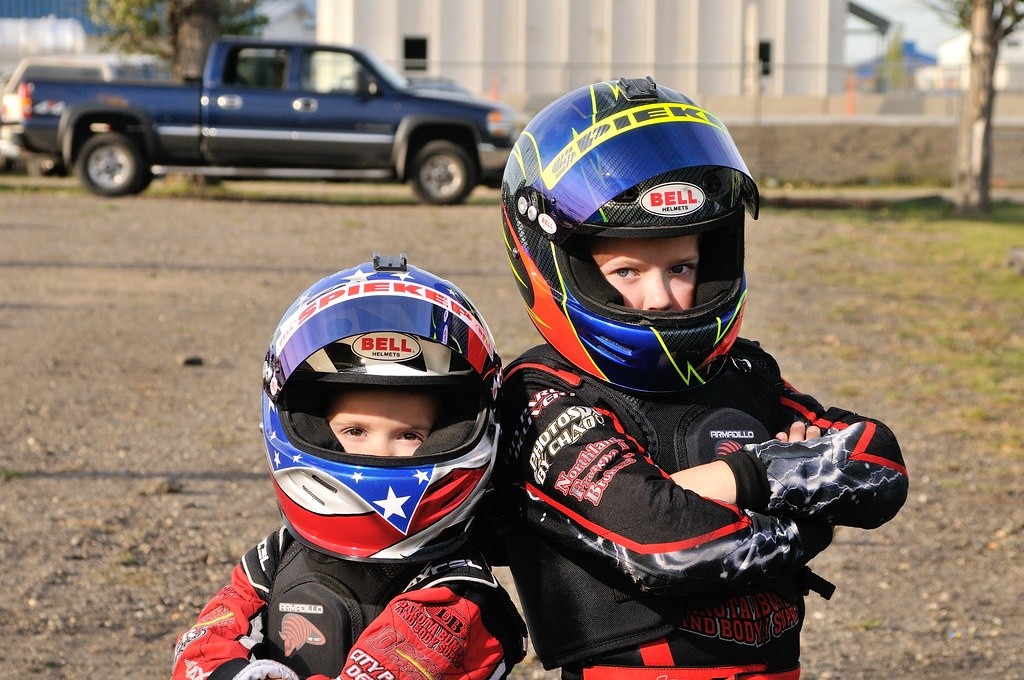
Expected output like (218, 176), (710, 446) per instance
(10, 34), (530, 206)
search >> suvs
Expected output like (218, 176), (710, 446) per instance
(1, 53), (179, 173)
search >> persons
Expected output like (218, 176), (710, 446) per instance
(498, 78), (910, 679)
(172, 255), (528, 679)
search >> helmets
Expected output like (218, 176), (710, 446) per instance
(501, 76), (760, 393)
(259, 250), (503, 565)
